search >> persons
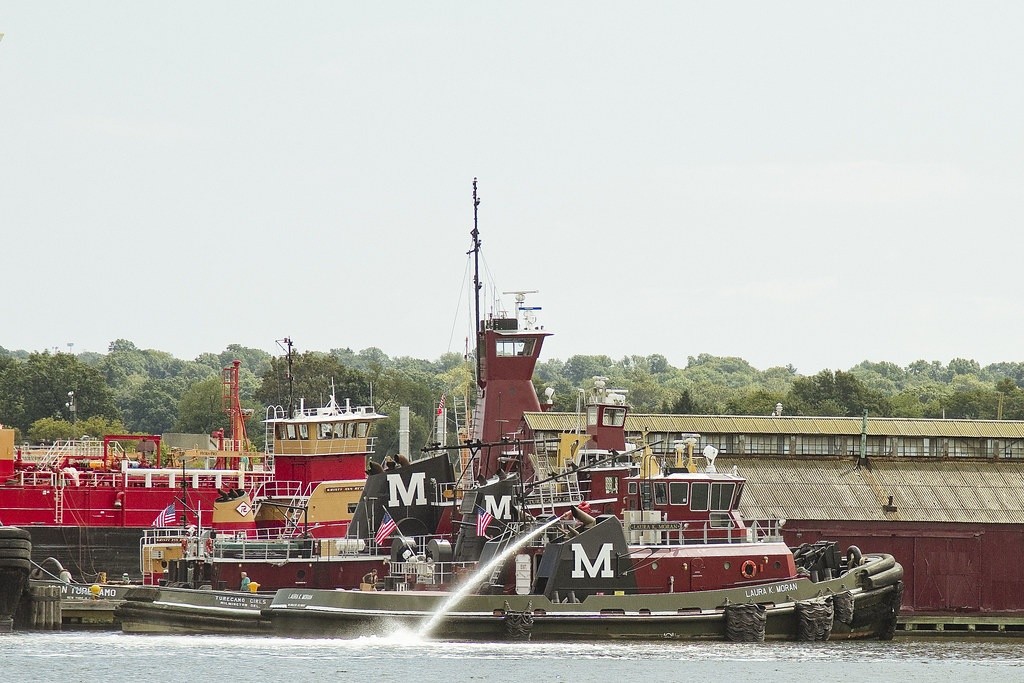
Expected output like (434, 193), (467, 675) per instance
(240, 571), (250, 591)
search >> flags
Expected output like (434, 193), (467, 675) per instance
(152, 502), (176, 527)
(477, 506), (494, 536)
(374, 512), (397, 546)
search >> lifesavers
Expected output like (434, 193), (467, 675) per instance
(181, 539), (188, 555)
(206, 538), (214, 554)
(740, 560), (757, 579)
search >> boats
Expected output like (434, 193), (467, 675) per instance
(114, 173), (904, 643)
(1, 335), (391, 582)
